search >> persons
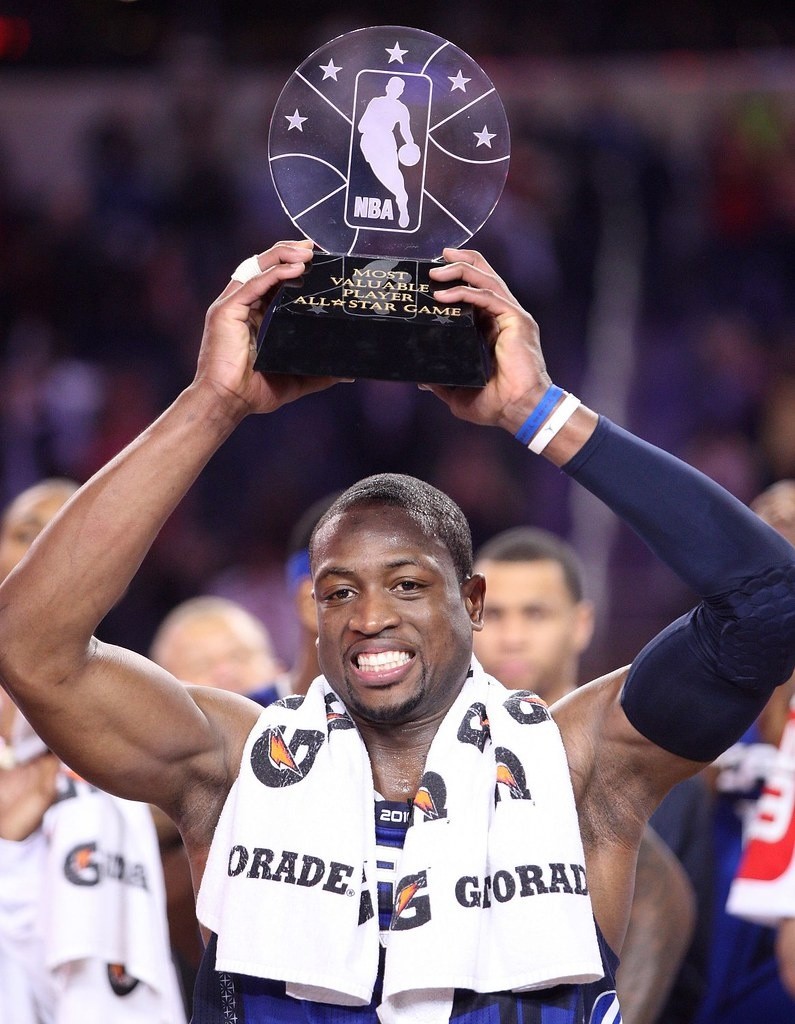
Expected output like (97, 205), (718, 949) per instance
(0, 243), (795, 1024)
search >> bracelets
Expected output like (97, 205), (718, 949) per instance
(528, 392), (582, 454)
(516, 384), (562, 444)
